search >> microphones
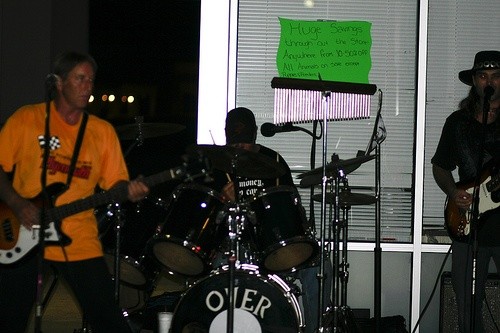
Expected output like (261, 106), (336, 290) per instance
(46, 73), (59, 88)
(483, 86), (494, 96)
(261, 122), (302, 137)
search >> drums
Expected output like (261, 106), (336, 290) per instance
(99, 196), (168, 290)
(167, 264), (304, 333)
(25, 249), (150, 333)
(243, 183), (318, 272)
(147, 181), (237, 276)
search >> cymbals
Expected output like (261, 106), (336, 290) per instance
(300, 157), (361, 188)
(115, 120), (186, 140)
(311, 191), (377, 206)
(295, 155), (375, 179)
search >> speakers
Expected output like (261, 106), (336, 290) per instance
(439, 271), (500, 332)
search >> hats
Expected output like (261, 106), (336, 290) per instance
(458, 51), (500, 86)
(224, 107), (257, 144)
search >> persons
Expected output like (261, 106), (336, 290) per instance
(431, 51), (500, 333)
(213, 107), (333, 333)
(0, 51), (149, 333)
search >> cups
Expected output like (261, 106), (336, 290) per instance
(159, 312), (172, 333)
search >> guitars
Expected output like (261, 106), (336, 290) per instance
(0, 149), (214, 264)
(444, 165), (500, 237)
